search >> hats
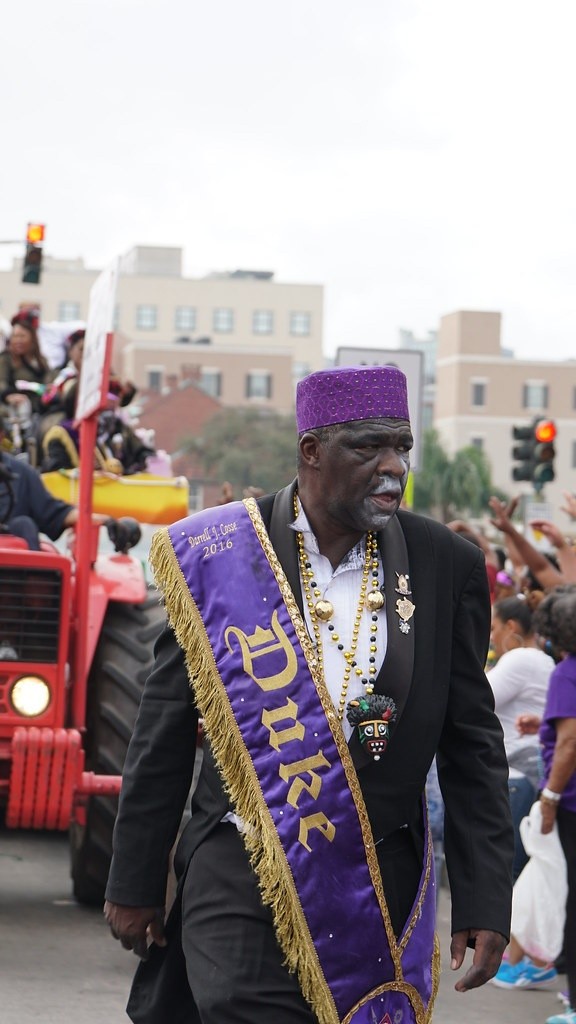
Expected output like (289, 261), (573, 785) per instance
(11, 311), (39, 331)
(64, 330), (85, 348)
(107, 380), (126, 403)
(296, 366), (410, 434)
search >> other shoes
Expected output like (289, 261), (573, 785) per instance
(557, 990), (570, 1007)
(546, 1007), (576, 1024)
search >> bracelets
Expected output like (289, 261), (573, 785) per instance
(555, 539), (567, 548)
(541, 797), (559, 806)
(541, 788), (561, 801)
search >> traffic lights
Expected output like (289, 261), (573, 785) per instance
(533, 415), (556, 483)
(21, 222), (45, 284)
(512, 424), (530, 482)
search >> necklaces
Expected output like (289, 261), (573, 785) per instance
(289, 488), (397, 766)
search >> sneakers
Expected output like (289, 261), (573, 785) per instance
(515, 959), (559, 989)
(487, 956), (522, 989)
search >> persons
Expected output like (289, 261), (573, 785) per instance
(102, 367), (514, 1024)
(0, 302), (265, 505)
(1, 416), (111, 551)
(426, 489), (576, 1024)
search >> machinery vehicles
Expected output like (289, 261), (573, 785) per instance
(0, 258), (190, 904)
